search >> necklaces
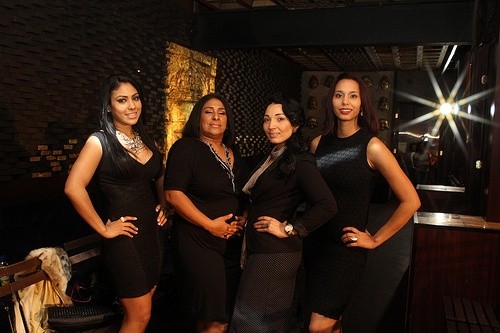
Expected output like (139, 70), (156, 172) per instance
(115, 130), (142, 154)
(209, 143), (236, 192)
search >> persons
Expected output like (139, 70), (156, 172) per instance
(406, 140), (438, 185)
(163, 94), (248, 333)
(231, 98), (338, 333)
(302, 73), (422, 333)
(64, 75), (170, 333)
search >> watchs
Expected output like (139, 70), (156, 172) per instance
(283, 222), (295, 236)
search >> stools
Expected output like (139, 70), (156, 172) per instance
(47, 303), (120, 333)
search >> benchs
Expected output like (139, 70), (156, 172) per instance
(442, 296), (500, 333)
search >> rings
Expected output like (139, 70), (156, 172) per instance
(349, 236), (357, 241)
(120, 217), (125, 222)
(261, 224), (268, 228)
